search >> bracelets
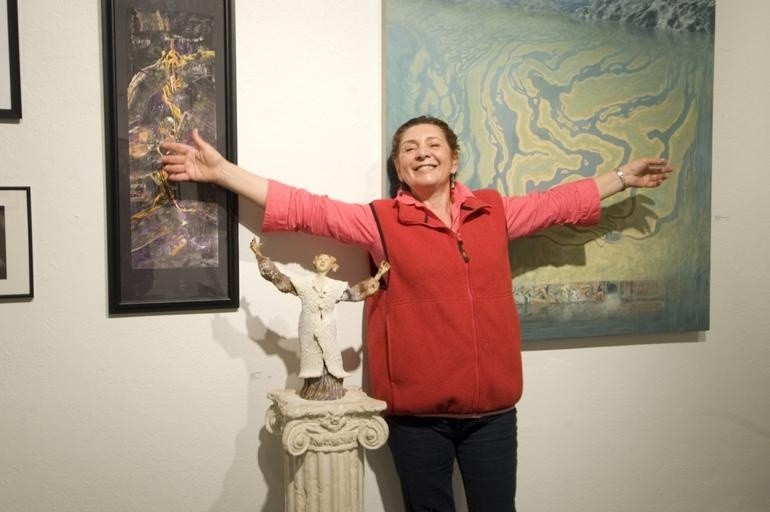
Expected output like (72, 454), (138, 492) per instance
(614, 168), (626, 185)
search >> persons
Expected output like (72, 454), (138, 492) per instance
(160, 114), (676, 511)
(248, 236), (392, 400)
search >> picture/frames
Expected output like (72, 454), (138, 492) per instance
(0, 0), (22, 118)
(0, 187), (34, 298)
(101, 0), (240, 314)
